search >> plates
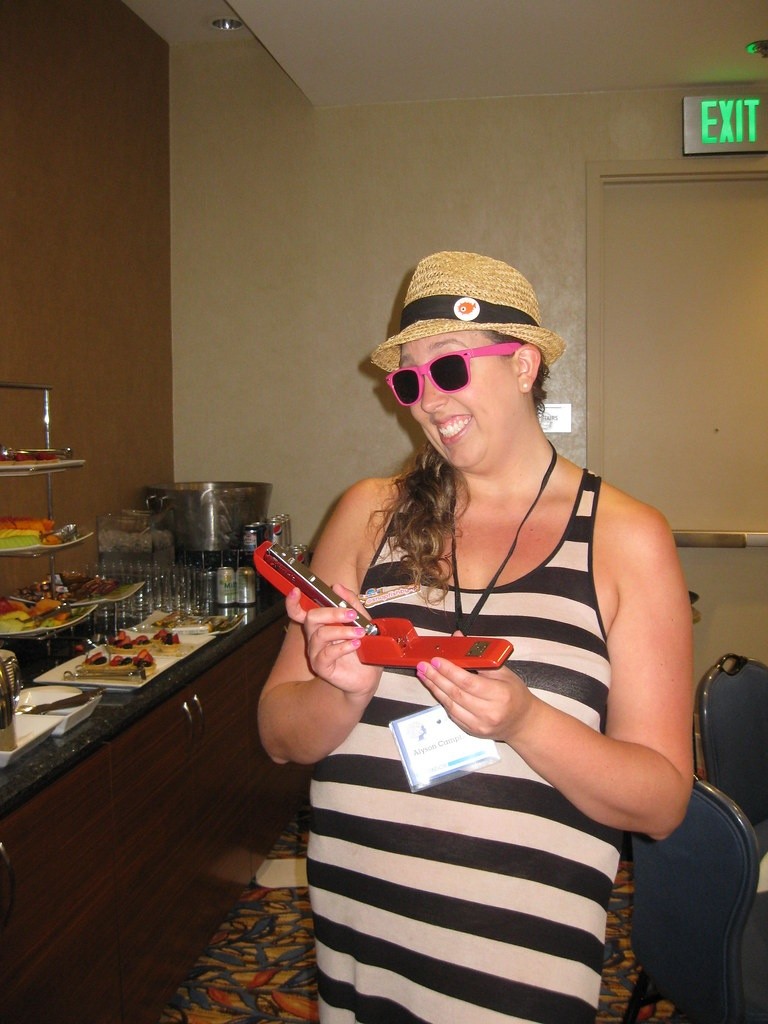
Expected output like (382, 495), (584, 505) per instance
(15, 686), (102, 736)
(0, 531), (96, 554)
(0, 605), (101, 635)
(0, 457), (87, 471)
(31, 608), (247, 692)
(10, 582), (147, 605)
(0, 713), (63, 770)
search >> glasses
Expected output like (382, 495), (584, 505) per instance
(385, 342), (522, 406)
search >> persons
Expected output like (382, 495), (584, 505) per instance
(254, 249), (693, 1024)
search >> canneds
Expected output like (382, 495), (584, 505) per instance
(216, 567), (235, 605)
(235, 567), (256, 604)
(242, 513), (309, 569)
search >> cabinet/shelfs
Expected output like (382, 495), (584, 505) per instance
(0, 612), (313, 1024)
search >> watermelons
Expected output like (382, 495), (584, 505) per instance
(0, 515), (56, 533)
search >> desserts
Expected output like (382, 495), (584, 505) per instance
(0, 453), (59, 466)
(81, 629), (181, 678)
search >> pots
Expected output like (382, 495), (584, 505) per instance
(142, 482), (273, 553)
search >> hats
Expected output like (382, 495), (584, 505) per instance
(371, 251), (567, 372)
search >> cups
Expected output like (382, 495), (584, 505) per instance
(75, 557), (215, 622)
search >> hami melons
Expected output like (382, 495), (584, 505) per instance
(7, 598), (71, 621)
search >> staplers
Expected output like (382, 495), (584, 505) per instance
(250, 537), (517, 672)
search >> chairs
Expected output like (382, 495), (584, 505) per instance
(692, 652), (768, 861)
(620, 775), (768, 1024)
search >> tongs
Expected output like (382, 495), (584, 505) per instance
(64, 664), (146, 683)
(23, 688), (102, 713)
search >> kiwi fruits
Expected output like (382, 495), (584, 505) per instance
(0, 534), (45, 549)
